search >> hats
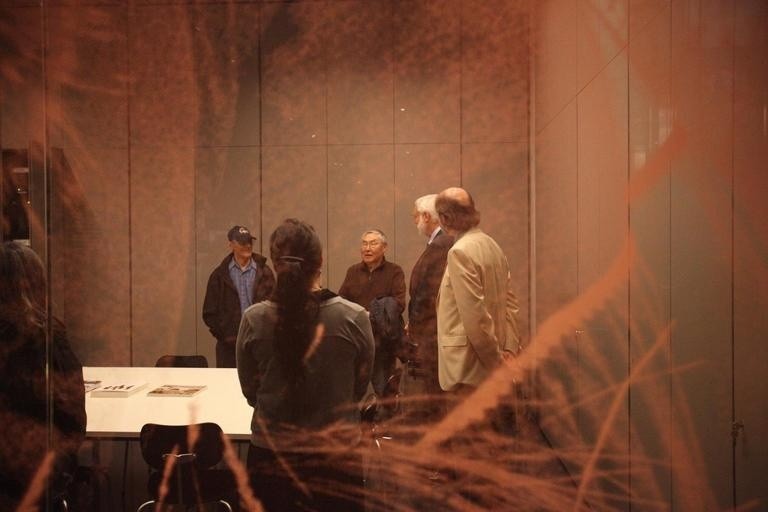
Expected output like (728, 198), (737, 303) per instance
(227, 225), (257, 243)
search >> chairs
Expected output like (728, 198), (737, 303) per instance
(78, 353), (254, 512)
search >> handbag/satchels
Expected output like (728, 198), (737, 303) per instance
(0, 238), (87, 436)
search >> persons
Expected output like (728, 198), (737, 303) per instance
(0, 240), (89, 512)
(0, 201), (28, 243)
(434, 185), (525, 500)
(403, 192), (458, 411)
(234, 217), (376, 512)
(335, 229), (409, 420)
(200, 224), (281, 367)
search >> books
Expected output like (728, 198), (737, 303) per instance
(91, 382), (149, 398)
(147, 383), (208, 399)
(83, 379), (102, 393)
